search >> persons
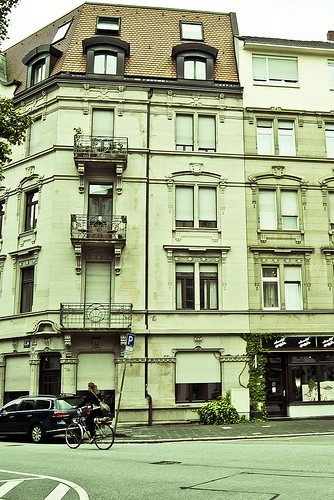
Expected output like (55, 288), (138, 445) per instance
(72, 381), (106, 444)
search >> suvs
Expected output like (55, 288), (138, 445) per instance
(0, 395), (85, 444)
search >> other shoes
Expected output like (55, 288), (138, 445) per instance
(89, 436), (95, 443)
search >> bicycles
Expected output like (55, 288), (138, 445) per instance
(65, 407), (115, 450)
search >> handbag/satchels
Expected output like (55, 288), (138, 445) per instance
(100, 401), (110, 412)
